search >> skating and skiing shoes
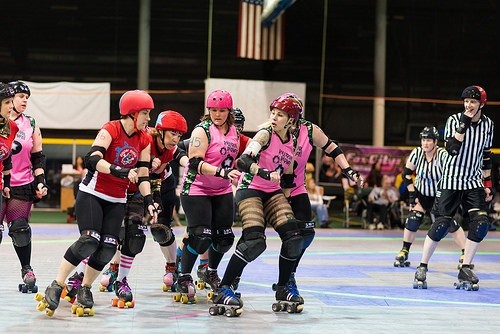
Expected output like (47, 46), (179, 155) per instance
(458, 255), (465, 268)
(162, 262), (178, 291)
(19, 264), (38, 293)
(207, 285), (243, 316)
(197, 269), (221, 292)
(71, 284), (95, 316)
(272, 282), (304, 313)
(231, 278), (241, 299)
(111, 276), (134, 308)
(272, 272), (300, 296)
(454, 265), (479, 291)
(100, 260), (119, 291)
(34, 280), (64, 316)
(173, 274), (198, 303)
(59, 271), (84, 303)
(177, 247), (184, 273)
(195, 263), (209, 285)
(393, 247), (411, 267)
(413, 266), (428, 289)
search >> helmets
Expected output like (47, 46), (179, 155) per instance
(8, 81), (30, 96)
(0, 82), (15, 100)
(461, 85), (486, 102)
(119, 90), (155, 115)
(206, 90), (233, 108)
(155, 110), (188, 133)
(420, 126), (439, 137)
(232, 108), (245, 125)
(270, 93), (304, 123)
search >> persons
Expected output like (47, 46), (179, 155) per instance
(210, 94), (305, 304)
(66, 155), (89, 222)
(487, 199), (500, 230)
(414, 86), (495, 281)
(396, 127), (468, 262)
(306, 177), (335, 228)
(1, 81), (49, 284)
(45, 89), (158, 308)
(0, 81), (20, 242)
(346, 154), (408, 230)
(177, 88), (241, 287)
(66, 110), (189, 301)
(99, 92), (364, 293)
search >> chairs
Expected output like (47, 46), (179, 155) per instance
(309, 186), (336, 224)
(342, 178), (367, 229)
